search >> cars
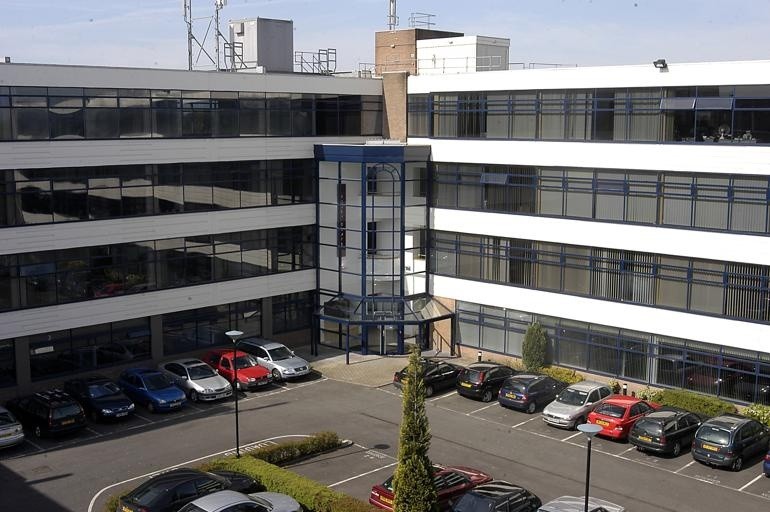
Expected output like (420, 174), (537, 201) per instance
(498, 372), (569, 413)
(15, 251), (156, 298)
(694, 414), (770, 472)
(628, 407), (708, 459)
(394, 361), (462, 401)
(555, 333), (770, 404)
(60, 371), (136, 425)
(0, 405), (25, 451)
(175, 489), (303, 512)
(202, 347), (273, 391)
(115, 469), (260, 512)
(588, 395), (660, 442)
(116, 367), (188, 414)
(157, 357), (234, 403)
(456, 361), (517, 401)
(369, 462), (628, 512)
(543, 380), (614, 430)
(763, 446), (770, 477)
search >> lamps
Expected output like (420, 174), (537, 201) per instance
(653, 58), (666, 68)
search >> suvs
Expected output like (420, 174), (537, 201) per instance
(7, 386), (88, 442)
(233, 336), (313, 383)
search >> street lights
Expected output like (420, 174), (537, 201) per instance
(577, 424), (604, 512)
(226, 328), (243, 459)
